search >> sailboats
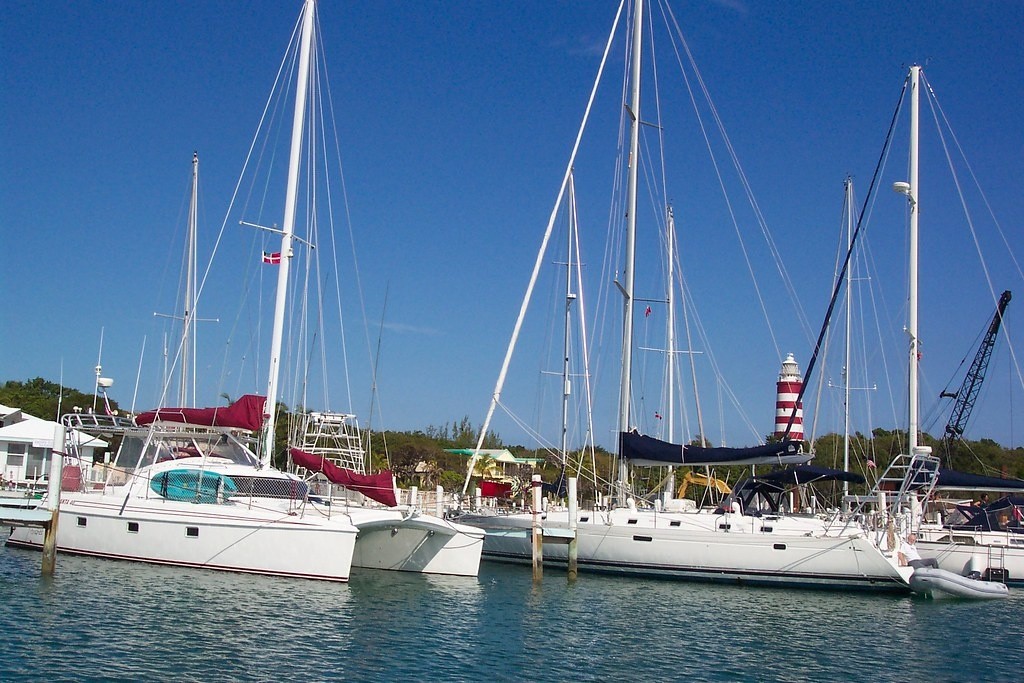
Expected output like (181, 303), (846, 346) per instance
(0, 0), (1024, 594)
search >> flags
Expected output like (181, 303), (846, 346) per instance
(263, 251), (281, 265)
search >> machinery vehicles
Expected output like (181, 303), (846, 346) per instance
(676, 470), (734, 500)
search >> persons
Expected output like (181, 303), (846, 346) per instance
(897, 533), (939, 569)
(934, 491), (1022, 532)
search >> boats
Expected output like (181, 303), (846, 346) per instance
(908, 567), (1011, 600)
